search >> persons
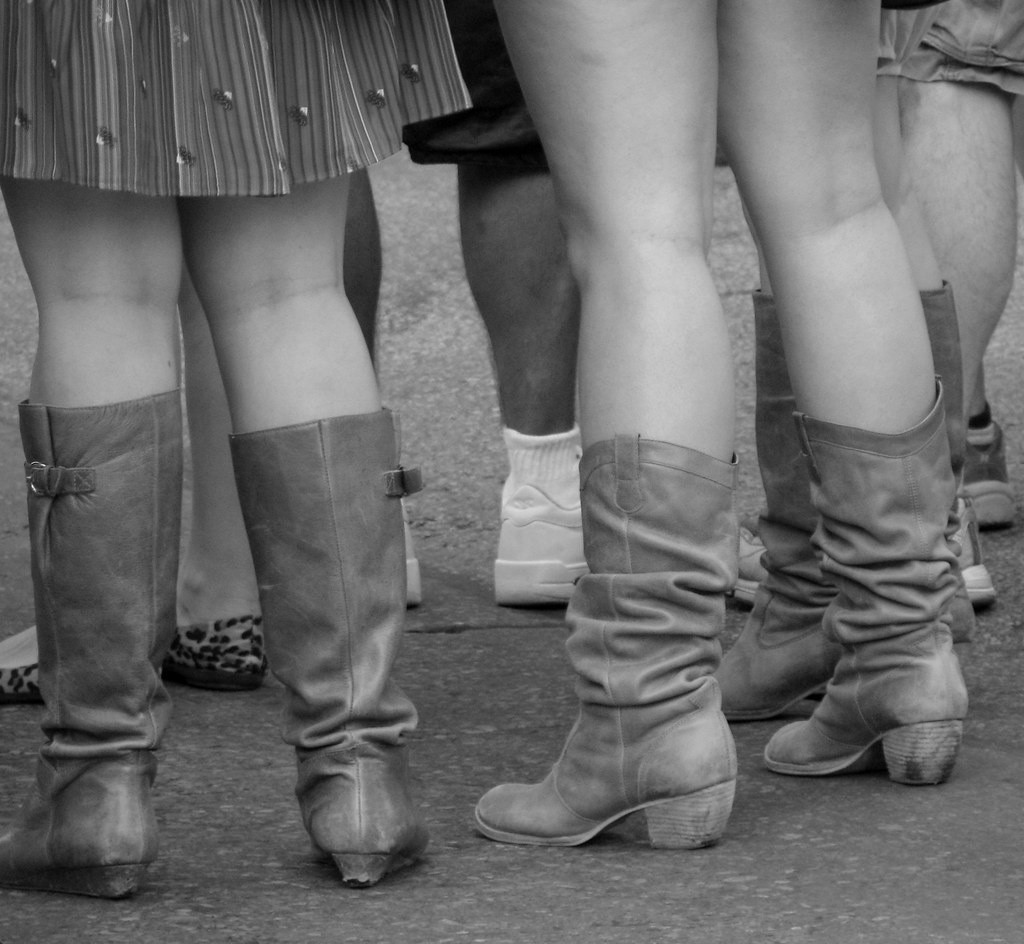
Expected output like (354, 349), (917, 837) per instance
(1, 0), (1024, 900)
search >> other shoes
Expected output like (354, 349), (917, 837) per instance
(0, 659), (41, 704)
(163, 614), (268, 694)
(944, 493), (993, 604)
(961, 423), (1014, 524)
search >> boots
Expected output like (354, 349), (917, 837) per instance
(761, 378), (968, 784)
(712, 291), (845, 721)
(473, 429), (741, 848)
(0, 386), (188, 898)
(916, 280), (974, 643)
(226, 403), (430, 888)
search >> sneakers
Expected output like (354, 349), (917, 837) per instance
(491, 483), (588, 602)
(396, 494), (422, 607)
